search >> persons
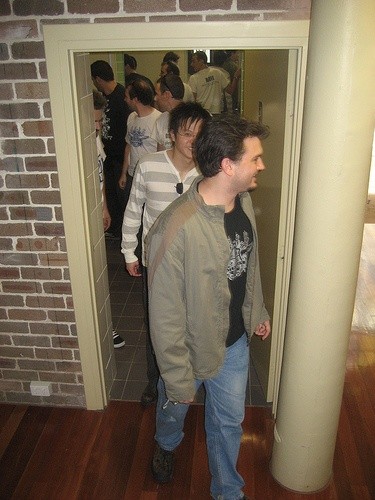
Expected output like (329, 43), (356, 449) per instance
(89, 50), (243, 234)
(144, 115), (271, 500)
(92, 89), (126, 349)
(121, 100), (213, 407)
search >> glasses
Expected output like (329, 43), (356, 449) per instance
(174, 182), (183, 195)
(162, 74), (174, 98)
(177, 129), (196, 139)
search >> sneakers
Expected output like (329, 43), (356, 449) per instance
(141, 374), (159, 407)
(152, 444), (176, 483)
(112, 329), (125, 348)
